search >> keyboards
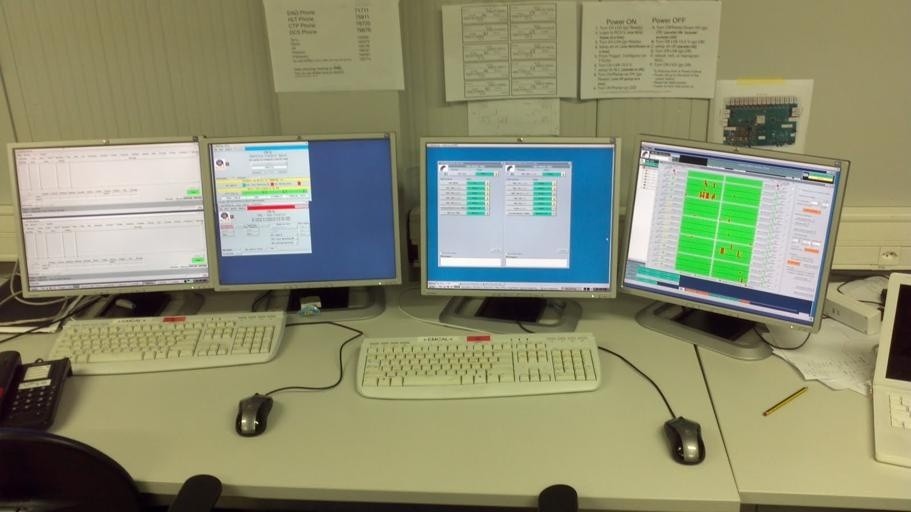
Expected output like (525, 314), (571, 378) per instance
(355, 331), (602, 401)
(45, 309), (286, 378)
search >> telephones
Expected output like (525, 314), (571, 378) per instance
(1, 350), (73, 431)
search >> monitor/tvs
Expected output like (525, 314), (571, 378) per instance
(615, 131), (852, 363)
(418, 135), (619, 337)
(5, 135), (214, 299)
(198, 130), (403, 292)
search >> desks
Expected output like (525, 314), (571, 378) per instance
(679, 267), (911, 512)
(2, 256), (741, 512)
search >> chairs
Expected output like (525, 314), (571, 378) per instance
(0, 424), (223, 512)
(538, 484), (578, 511)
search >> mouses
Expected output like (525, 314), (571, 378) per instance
(234, 391), (274, 438)
(664, 415), (706, 466)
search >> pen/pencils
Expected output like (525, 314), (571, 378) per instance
(765, 385), (809, 416)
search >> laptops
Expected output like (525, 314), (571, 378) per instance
(872, 272), (911, 468)
